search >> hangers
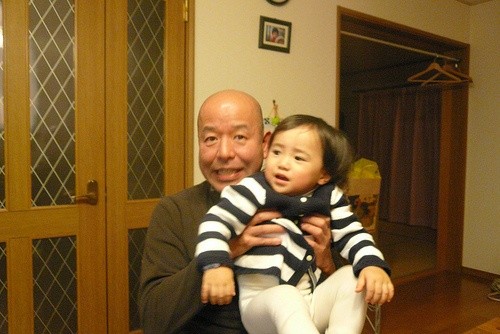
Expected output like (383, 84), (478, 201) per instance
(406, 51), (474, 90)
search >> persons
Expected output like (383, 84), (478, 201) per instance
(138, 88), (345, 334)
(191, 111), (396, 334)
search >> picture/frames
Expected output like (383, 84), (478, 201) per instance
(258, 15), (292, 54)
